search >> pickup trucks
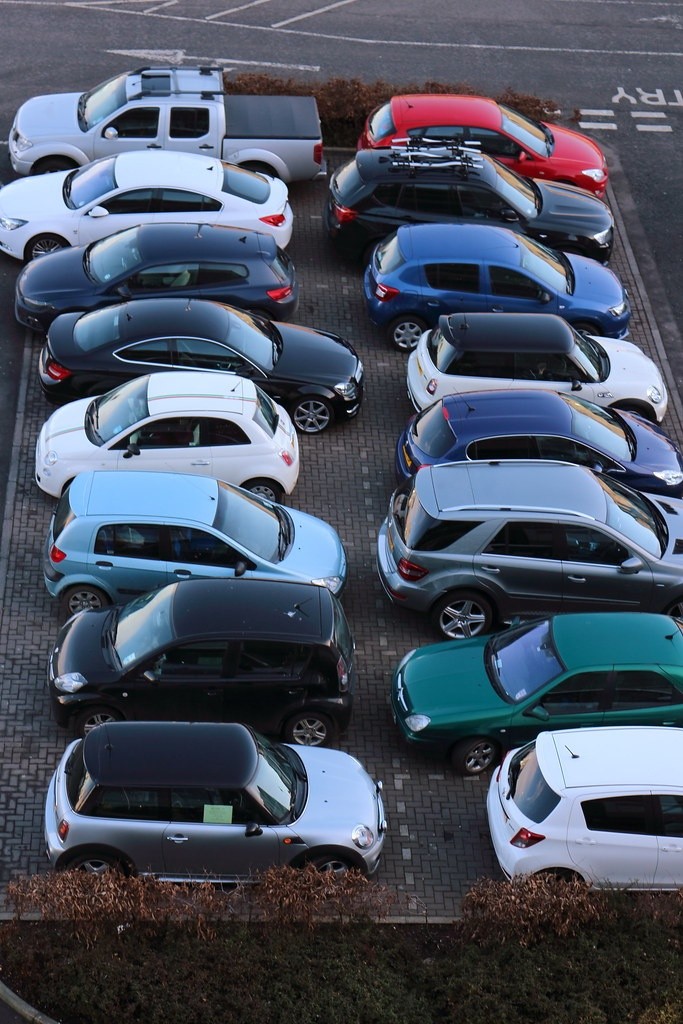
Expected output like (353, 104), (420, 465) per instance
(11, 64), (330, 189)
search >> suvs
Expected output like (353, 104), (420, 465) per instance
(323, 136), (616, 268)
(46, 576), (357, 749)
(374, 458), (683, 641)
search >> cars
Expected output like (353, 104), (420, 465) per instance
(405, 312), (669, 427)
(363, 222), (634, 355)
(393, 387), (683, 503)
(32, 371), (301, 505)
(35, 295), (368, 437)
(356, 92), (610, 202)
(44, 468), (351, 626)
(0, 149), (296, 270)
(386, 612), (683, 778)
(486, 726), (683, 899)
(14, 222), (301, 337)
(45, 720), (388, 906)
